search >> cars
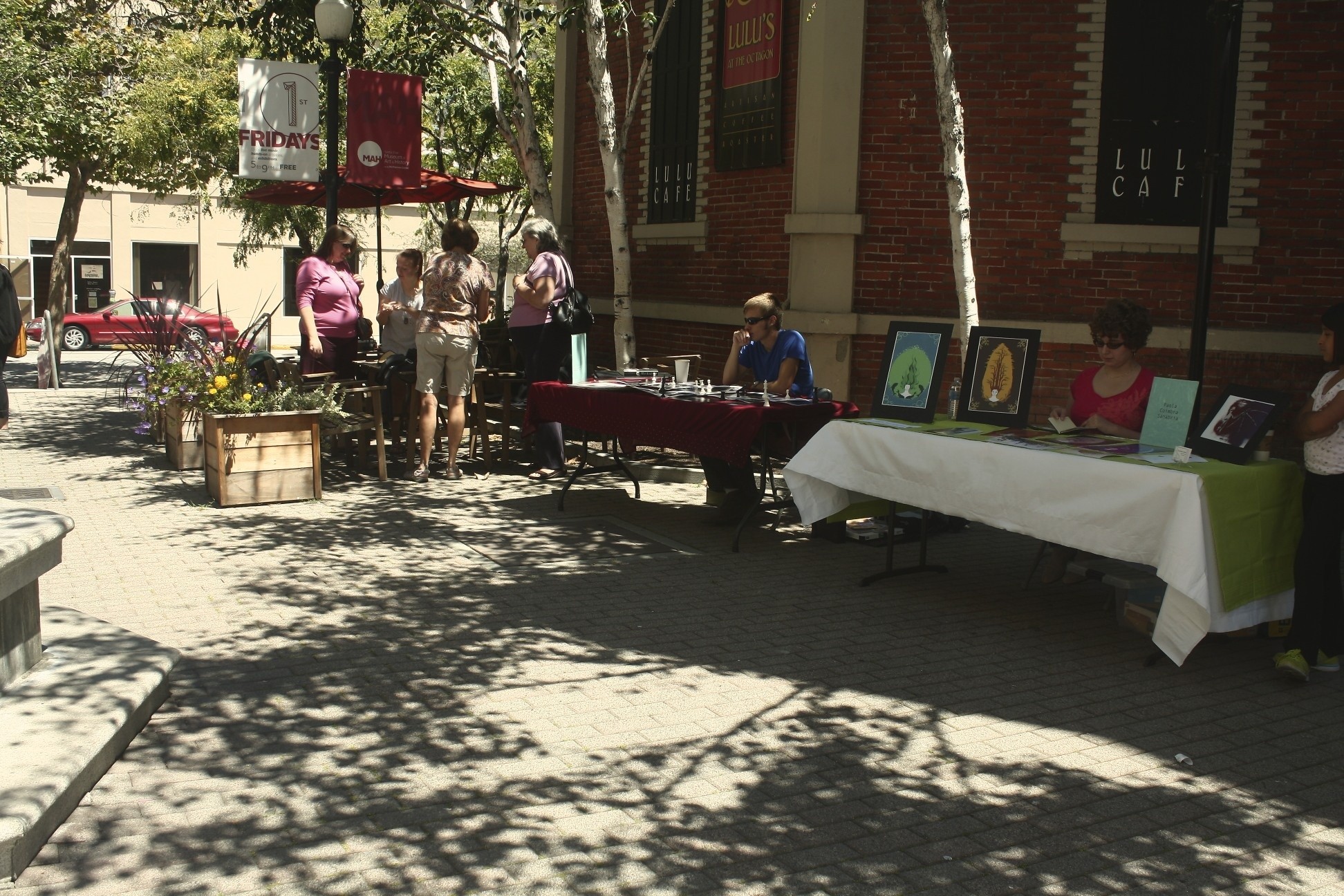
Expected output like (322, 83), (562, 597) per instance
(24, 297), (240, 351)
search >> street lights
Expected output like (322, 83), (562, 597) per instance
(314, 0), (356, 242)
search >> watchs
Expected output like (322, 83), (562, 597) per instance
(752, 381), (760, 392)
(405, 305), (409, 311)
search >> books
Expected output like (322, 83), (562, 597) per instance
(592, 367), (672, 380)
(1048, 416), (1097, 435)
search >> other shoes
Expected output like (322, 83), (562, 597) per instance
(329, 440), (360, 454)
(404, 441), (408, 453)
(702, 490), (761, 526)
(0, 428), (9, 437)
(1061, 555), (1106, 584)
(1041, 545), (1078, 584)
(389, 442), (400, 453)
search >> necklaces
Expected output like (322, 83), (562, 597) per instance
(1105, 363), (1139, 379)
(402, 288), (416, 324)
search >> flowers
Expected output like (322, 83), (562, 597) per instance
(104, 267), (353, 446)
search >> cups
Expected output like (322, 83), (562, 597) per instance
(1253, 430), (1274, 461)
(674, 360), (691, 383)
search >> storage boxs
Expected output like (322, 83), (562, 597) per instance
(1067, 560), (1168, 636)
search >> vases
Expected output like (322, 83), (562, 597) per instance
(144, 374), (165, 445)
(201, 407), (323, 507)
(164, 401), (205, 471)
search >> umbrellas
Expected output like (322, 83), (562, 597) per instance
(239, 161), (524, 343)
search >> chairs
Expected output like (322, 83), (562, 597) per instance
(263, 359), (387, 481)
(640, 354), (702, 381)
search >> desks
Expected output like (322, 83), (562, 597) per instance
(522, 378), (859, 554)
(353, 360), (525, 481)
(781, 414), (1305, 668)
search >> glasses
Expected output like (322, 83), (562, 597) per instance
(744, 315), (772, 325)
(1095, 339), (1126, 349)
(336, 240), (353, 250)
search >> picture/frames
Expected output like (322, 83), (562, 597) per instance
(1184, 383), (1293, 465)
(956, 326), (1041, 429)
(870, 320), (954, 423)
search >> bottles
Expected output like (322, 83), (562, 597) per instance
(947, 378), (962, 419)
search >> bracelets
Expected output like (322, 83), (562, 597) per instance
(515, 283), (523, 292)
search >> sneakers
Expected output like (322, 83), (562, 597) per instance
(1276, 647), (1308, 682)
(1272, 648), (1341, 672)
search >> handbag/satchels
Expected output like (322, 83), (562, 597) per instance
(357, 314), (373, 341)
(525, 249), (595, 334)
(5, 322), (27, 358)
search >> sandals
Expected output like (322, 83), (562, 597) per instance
(404, 466), (430, 483)
(446, 465), (463, 480)
(526, 462), (542, 474)
(529, 464), (567, 480)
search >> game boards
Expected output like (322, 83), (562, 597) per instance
(632, 372), (832, 413)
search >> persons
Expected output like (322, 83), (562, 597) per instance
(1275, 300), (1344, 682)
(375, 249), (423, 454)
(1041, 296), (1159, 586)
(700, 292), (814, 524)
(508, 216), (573, 479)
(296, 224), (371, 454)
(0, 263), (23, 431)
(401, 218), (496, 483)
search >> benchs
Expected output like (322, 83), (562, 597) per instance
(0, 497), (75, 691)
